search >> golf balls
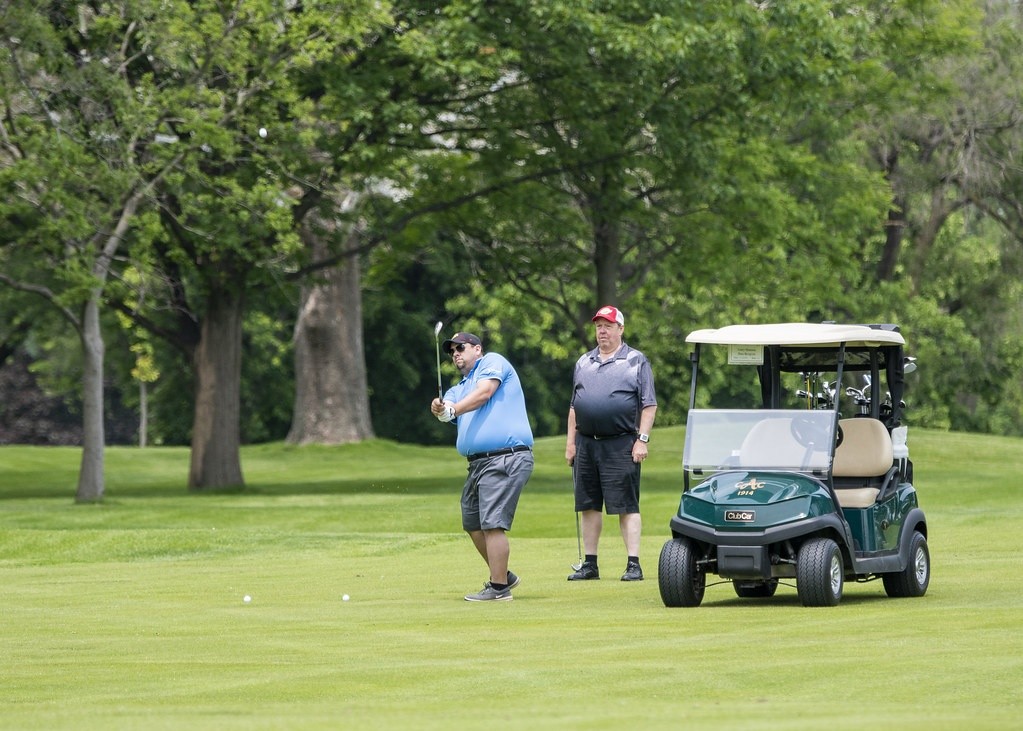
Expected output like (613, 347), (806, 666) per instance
(341, 594), (350, 601)
(243, 595), (251, 602)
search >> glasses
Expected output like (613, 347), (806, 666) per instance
(448, 343), (465, 357)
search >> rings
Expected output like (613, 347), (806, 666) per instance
(643, 458), (645, 461)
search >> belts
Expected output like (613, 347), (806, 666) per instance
(580, 431), (631, 440)
(467, 446), (530, 462)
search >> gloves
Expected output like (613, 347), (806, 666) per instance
(438, 406), (456, 423)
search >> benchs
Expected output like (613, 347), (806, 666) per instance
(737, 418), (893, 477)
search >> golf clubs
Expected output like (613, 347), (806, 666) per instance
(433, 321), (444, 404)
(794, 355), (918, 416)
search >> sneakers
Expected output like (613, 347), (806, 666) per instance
(489, 571), (521, 590)
(568, 561), (600, 580)
(464, 582), (512, 602)
(621, 561), (643, 581)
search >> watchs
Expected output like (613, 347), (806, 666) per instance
(637, 434), (650, 443)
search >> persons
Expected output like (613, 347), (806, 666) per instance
(565, 306), (658, 581)
(431, 331), (534, 602)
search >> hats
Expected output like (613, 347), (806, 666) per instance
(592, 306), (625, 325)
(442, 333), (482, 354)
